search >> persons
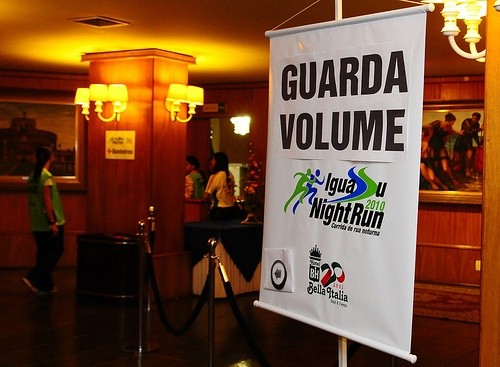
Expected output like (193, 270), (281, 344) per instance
(20, 146), (66, 295)
(203, 152), (242, 221)
(420, 111), (481, 190)
(185, 154), (207, 198)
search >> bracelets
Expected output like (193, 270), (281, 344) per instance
(49, 221), (56, 225)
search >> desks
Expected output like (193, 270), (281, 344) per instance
(76, 234), (148, 308)
(186, 221), (262, 297)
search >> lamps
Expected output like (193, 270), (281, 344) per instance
(164, 83), (204, 122)
(421, 0), (488, 62)
(75, 83), (128, 122)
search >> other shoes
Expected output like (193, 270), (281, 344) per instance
(22, 276), (37, 291)
(36, 287), (59, 295)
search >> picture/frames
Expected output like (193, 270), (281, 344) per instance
(0, 91), (86, 193)
(418, 99), (485, 204)
